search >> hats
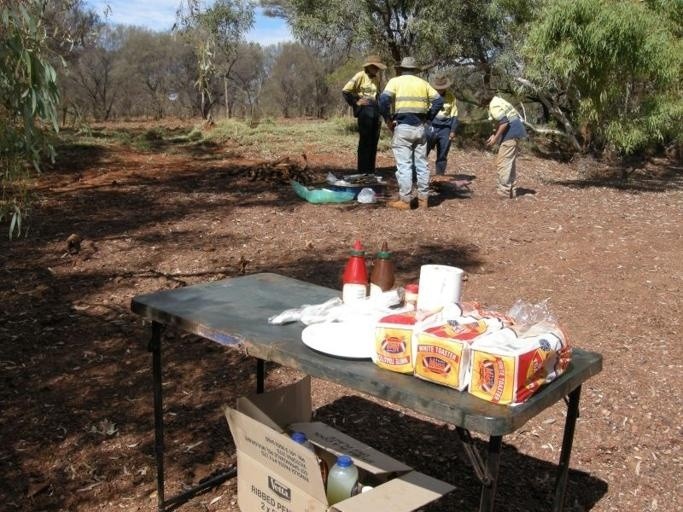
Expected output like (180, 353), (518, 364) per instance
(399, 56), (422, 71)
(429, 71), (450, 89)
(362, 54), (387, 70)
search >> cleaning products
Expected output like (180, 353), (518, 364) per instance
(327, 453), (359, 502)
(292, 433), (313, 452)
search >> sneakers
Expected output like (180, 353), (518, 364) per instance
(385, 197), (410, 210)
(417, 198), (427, 208)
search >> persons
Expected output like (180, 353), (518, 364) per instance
(474, 90), (526, 199)
(379, 54), (444, 212)
(411, 72), (458, 184)
(340, 53), (385, 176)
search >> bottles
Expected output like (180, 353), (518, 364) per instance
(369, 240), (395, 299)
(292, 432), (404, 506)
(340, 238), (367, 303)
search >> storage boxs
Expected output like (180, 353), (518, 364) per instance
(219, 375), (458, 511)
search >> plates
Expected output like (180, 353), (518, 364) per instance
(300, 320), (372, 361)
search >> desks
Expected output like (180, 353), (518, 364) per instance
(129, 272), (602, 511)
